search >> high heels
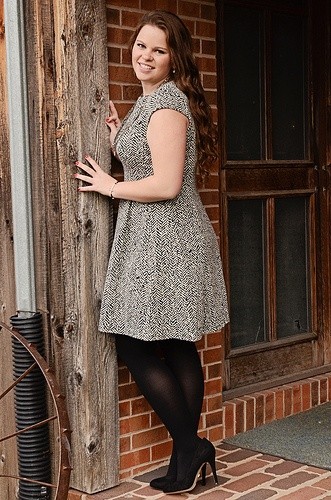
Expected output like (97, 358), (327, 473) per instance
(150, 437), (206, 489)
(163, 437), (218, 495)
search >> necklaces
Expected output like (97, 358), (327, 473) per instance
(112, 78), (168, 153)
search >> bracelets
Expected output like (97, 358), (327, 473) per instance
(110, 181), (118, 198)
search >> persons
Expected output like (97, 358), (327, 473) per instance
(71, 13), (231, 495)
